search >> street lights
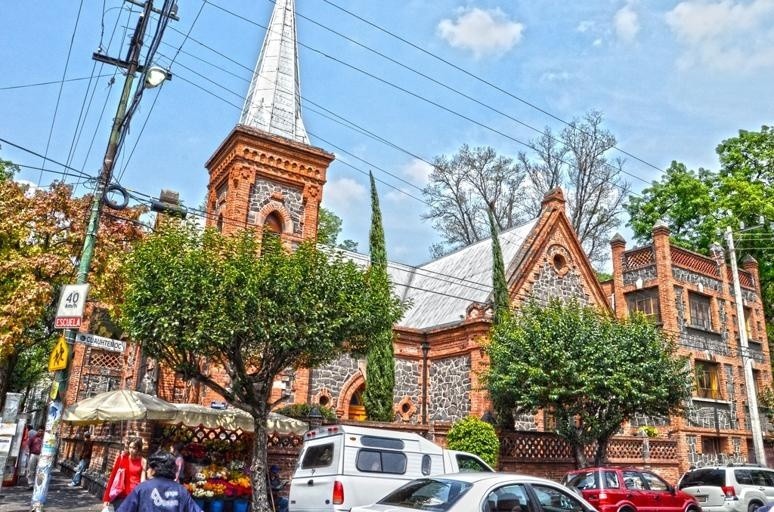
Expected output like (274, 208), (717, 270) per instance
(725, 216), (766, 466)
(30, 66), (169, 512)
(418, 332), (430, 421)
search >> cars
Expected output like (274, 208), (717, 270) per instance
(349, 472), (600, 512)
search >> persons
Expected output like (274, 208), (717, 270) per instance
(267, 464), (288, 512)
(67, 434), (92, 487)
(115, 451), (205, 512)
(170, 439), (186, 485)
(101, 436), (148, 511)
(17, 425), (45, 490)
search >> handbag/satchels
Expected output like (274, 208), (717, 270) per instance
(109, 467), (124, 502)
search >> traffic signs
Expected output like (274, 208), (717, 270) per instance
(56, 285), (86, 317)
(55, 318), (81, 328)
(76, 333), (125, 353)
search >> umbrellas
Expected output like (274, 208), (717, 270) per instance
(62, 388), (179, 456)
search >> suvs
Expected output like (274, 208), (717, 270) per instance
(675, 463), (774, 512)
(552, 465), (702, 512)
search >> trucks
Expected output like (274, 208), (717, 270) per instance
(286, 425), (551, 512)
